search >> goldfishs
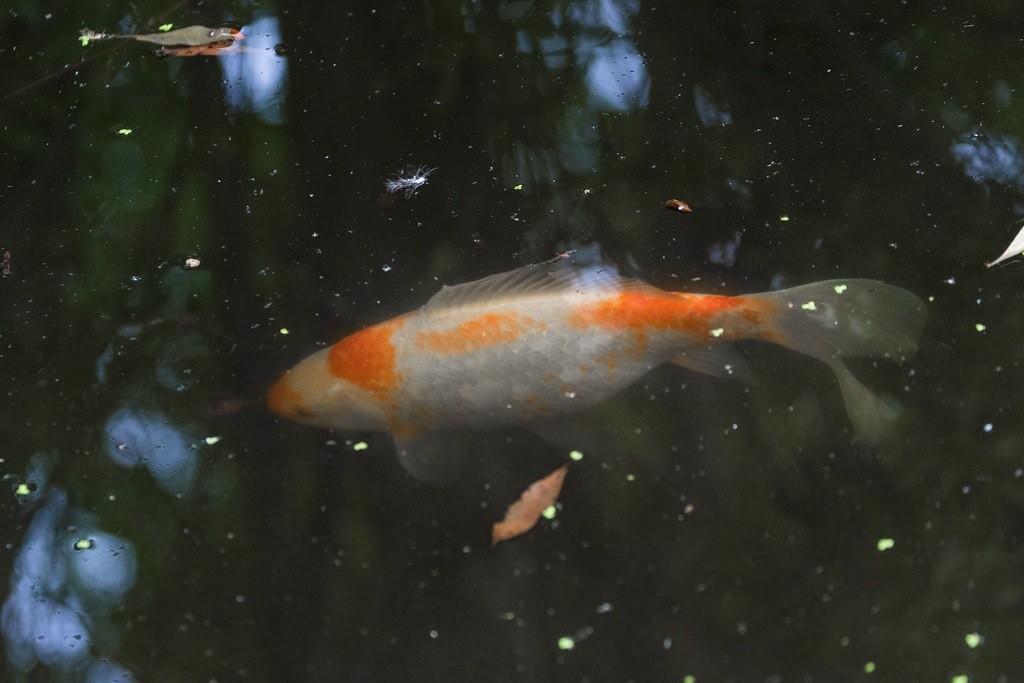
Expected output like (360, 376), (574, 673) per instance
(264, 242), (927, 484)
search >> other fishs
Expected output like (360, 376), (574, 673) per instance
(952, 132), (1024, 188)
(79, 25), (275, 57)
